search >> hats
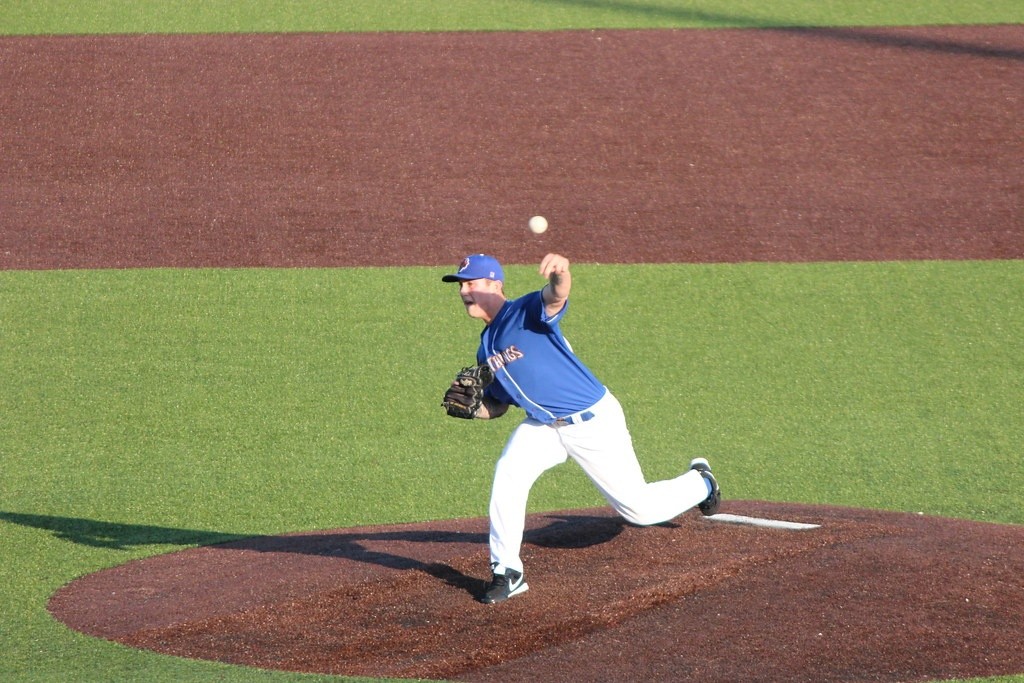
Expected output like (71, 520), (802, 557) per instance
(442, 253), (504, 284)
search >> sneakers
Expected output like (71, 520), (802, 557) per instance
(690, 457), (721, 516)
(480, 567), (529, 604)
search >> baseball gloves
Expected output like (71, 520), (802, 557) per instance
(440, 364), (495, 420)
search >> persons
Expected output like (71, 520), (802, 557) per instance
(438, 252), (720, 607)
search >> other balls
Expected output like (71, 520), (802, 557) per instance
(528, 215), (549, 235)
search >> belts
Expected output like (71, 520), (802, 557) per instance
(547, 412), (595, 428)
